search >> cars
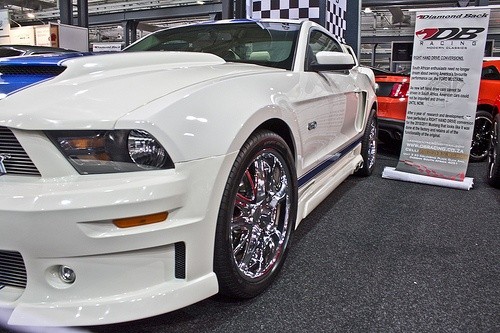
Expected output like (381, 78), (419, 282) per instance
(359, 56), (500, 163)
(0, 44), (95, 103)
(0, 16), (379, 333)
